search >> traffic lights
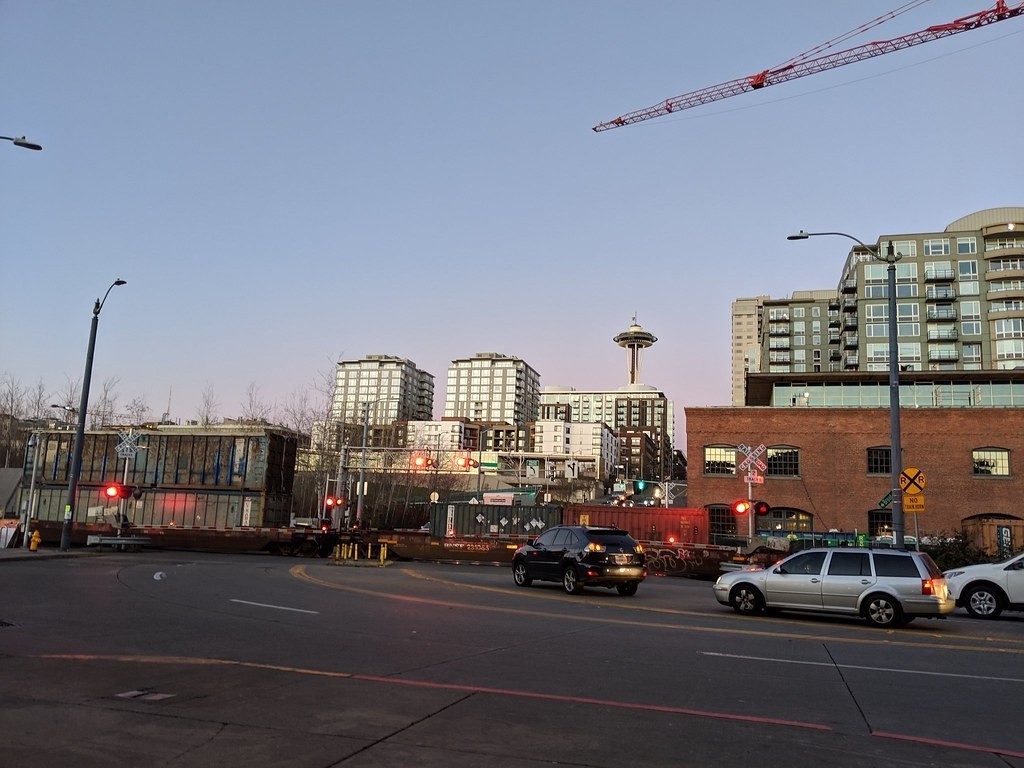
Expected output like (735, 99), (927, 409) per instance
(458, 458), (474, 466)
(336, 499), (342, 506)
(105, 487), (118, 497)
(326, 499), (333, 506)
(755, 502), (770, 516)
(416, 458), (433, 467)
(734, 501), (747, 515)
(639, 482), (643, 489)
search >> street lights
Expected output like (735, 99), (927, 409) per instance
(357, 398), (395, 526)
(787, 230), (906, 550)
(23, 434), (42, 548)
(59, 280), (127, 551)
(477, 427), (499, 501)
(571, 447), (593, 504)
(434, 432), (449, 492)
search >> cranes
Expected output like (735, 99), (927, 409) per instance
(592, 0), (1024, 133)
(74, 408), (157, 420)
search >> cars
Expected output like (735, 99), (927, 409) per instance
(942, 551), (1024, 620)
(421, 522), (430, 532)
(644, 496), (656, 507)
(601, 496), (620, 506)
(712, 546), (956, 630)
(636, 503), (647, 507)
(622, 500), (634, 507)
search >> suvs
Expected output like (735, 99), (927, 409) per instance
(512, 524), (647, 598)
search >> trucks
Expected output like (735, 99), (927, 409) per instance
(612, 482), (635, 501)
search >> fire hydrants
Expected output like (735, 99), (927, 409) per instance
(30, 530), (42, 552)
